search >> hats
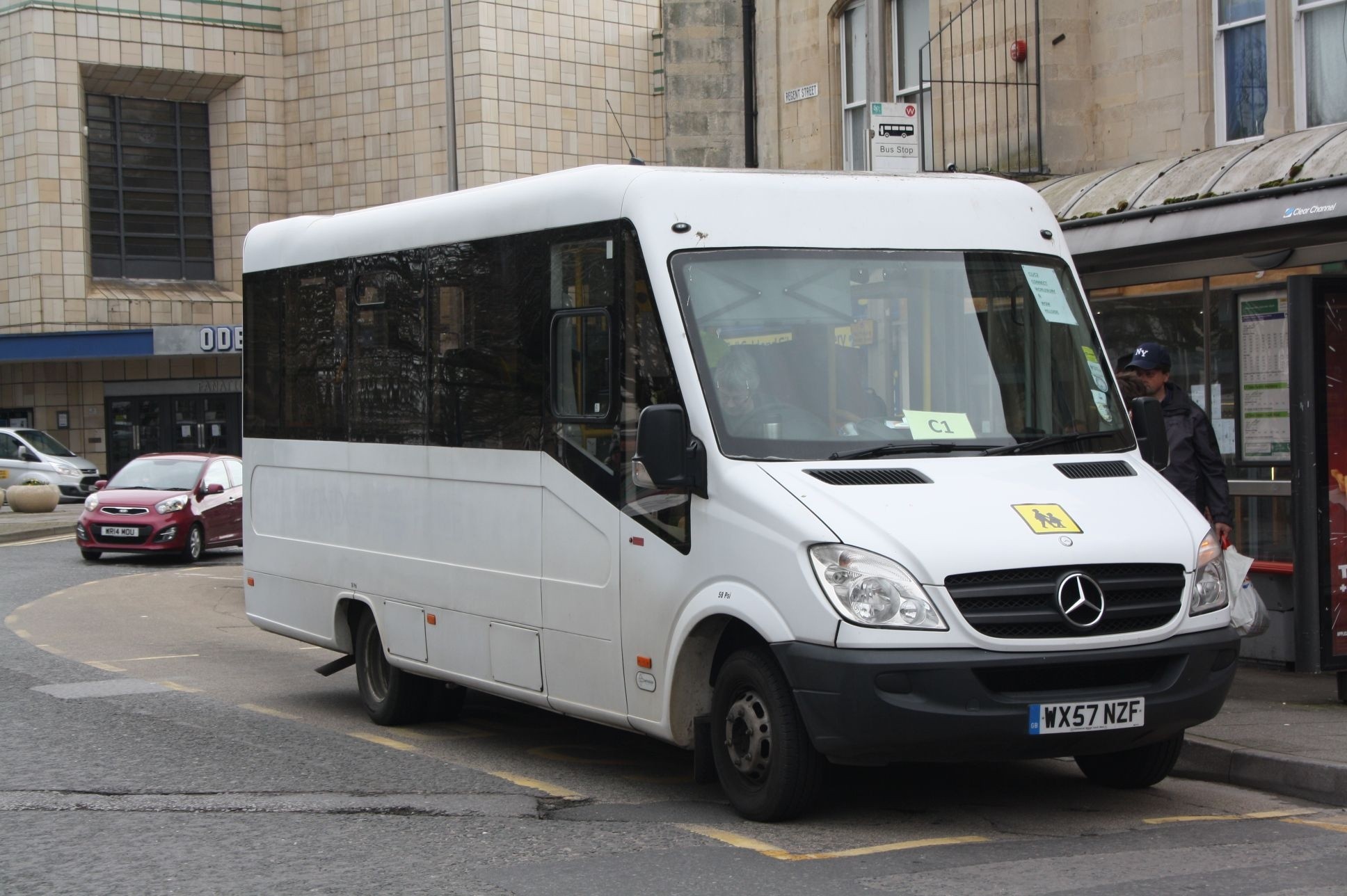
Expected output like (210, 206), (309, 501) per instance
(1124, 343), (1171, 371)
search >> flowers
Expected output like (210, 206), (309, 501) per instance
(20, 478), (42, 486)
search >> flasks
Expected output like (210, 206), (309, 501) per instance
(762, 414), (783, 440)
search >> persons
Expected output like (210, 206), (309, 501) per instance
(711, 355), (798, 442)
(1086, 341), (1235, 549)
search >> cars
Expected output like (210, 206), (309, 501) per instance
(75, 451), (243, 566)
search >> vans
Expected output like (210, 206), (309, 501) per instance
(243, 164), (1242, 826)
(0, 427), (101, 502)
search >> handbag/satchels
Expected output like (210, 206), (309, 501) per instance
(1221, 533), (1271, 639)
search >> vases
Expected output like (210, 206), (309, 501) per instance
(6, 485), (60, 512)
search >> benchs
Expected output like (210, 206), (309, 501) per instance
(760, 341), (868, 421)
